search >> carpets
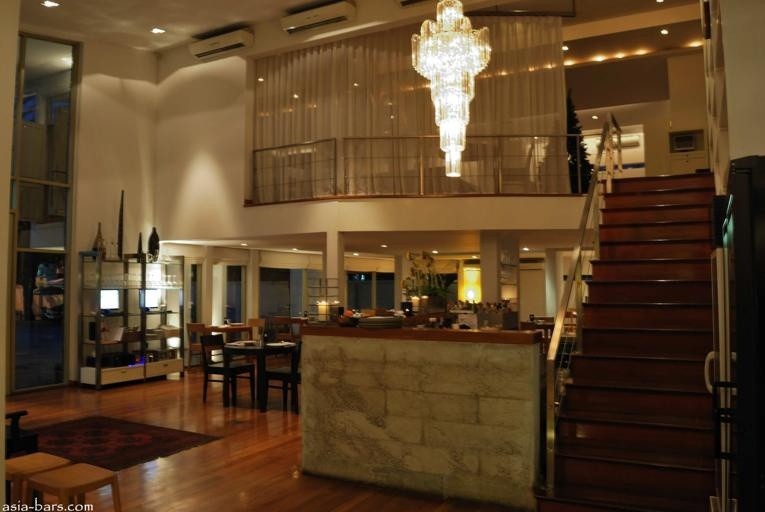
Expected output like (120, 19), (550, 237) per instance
(27, 415), (222, 472)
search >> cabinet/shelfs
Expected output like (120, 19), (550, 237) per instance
(77, 250), (185, 390)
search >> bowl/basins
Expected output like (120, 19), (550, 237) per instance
(336, 318), (360, 327)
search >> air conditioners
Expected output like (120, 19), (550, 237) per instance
(279, 1), (357, 37)
(394, 0), (436, 9)
(187, 29), (255, 61)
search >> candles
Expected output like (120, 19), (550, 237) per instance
(317, 301), (329, 321)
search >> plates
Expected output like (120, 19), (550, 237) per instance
(358, 316), (402, 328)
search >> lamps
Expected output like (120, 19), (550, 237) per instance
(409, 1), (492, 179)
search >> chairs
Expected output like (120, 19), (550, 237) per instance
(187, 315), (318, 416)
(6, 410), (41, 512)
(520, 317), (554, 353)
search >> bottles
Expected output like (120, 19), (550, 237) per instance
(263, 320), (271, 341)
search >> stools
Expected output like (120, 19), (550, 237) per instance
(26, 462), (124, 511)
(6, 451), (73, 507)
